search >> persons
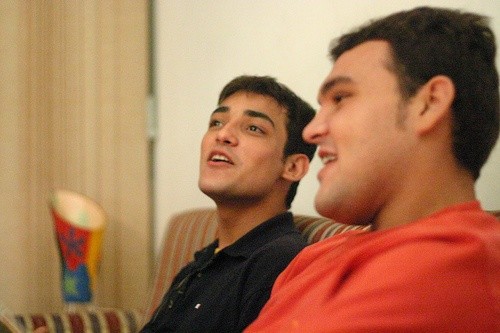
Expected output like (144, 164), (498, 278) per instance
(240, 7), (499, 332)
(137, 74), (317, 333)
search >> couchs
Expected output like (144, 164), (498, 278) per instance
(0, 206), (372, 333)
